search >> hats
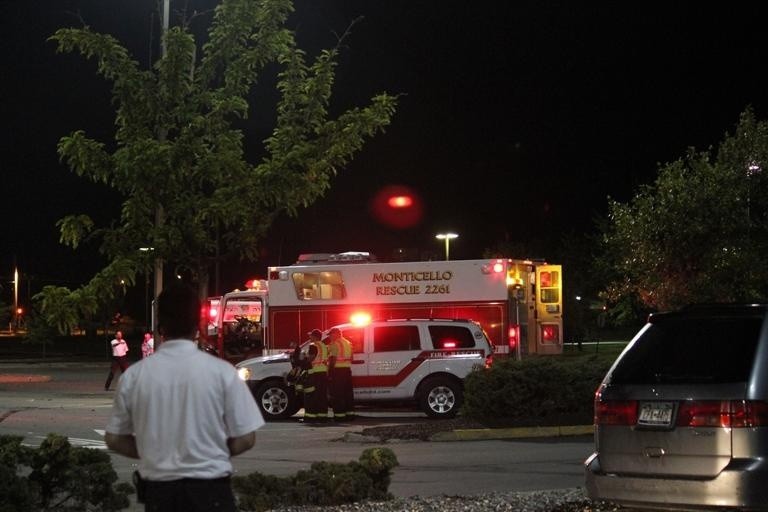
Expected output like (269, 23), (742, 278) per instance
(307, 329), (322, 337)
(326, 328), (341, 335)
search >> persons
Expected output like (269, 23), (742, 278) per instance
(105, 330), (130, 390)
(141, 332), (154, 357)
(298, 329), (328, 424)
(326, 328), (355, 421)
(104, 283), (266, 512)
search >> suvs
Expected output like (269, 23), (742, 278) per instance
(233, 317), (493, 418)
(582, 301), (768, 512)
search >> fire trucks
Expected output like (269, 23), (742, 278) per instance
(216, 258), (563, 365)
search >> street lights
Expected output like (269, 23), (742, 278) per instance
(436, 232), (458, 261)
